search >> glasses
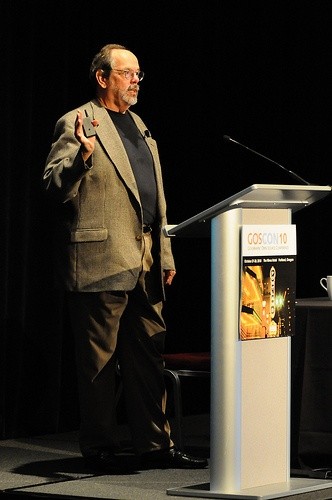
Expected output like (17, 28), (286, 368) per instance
(112, 68), (145, 81)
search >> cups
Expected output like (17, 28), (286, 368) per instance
(320, 276), (332, 300)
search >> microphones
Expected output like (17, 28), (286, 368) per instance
(224, 135), (309, 185)
(145, 130), (149, 138)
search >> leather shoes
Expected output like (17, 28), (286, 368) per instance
(87, 452), (134, 476)
(148, 447), (210, 469)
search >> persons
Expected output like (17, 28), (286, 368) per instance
(44, 43), (208, 476)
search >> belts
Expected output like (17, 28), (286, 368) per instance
(143, 225), (159, 233)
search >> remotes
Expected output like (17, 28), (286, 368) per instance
(82, 116), (96, 137)
